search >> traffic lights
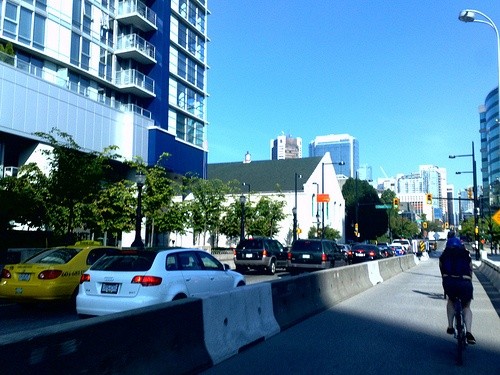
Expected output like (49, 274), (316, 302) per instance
(423, 222), (427, 229)
(427, 194), (432, 205)
(444, 221), (448, 228)
(467, 188), (472, 199)
(393, 197), (400, 209)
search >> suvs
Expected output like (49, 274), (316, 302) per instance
(288, 239), (349, 273)
(233, 238), (289, 274)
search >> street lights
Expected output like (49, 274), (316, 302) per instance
(448, 141), (480, 260)
(354, 171), (373, 197)
(242, 182), (251, 199)
(321, 161), (345, 227)
(292, 171), (303, 240)
(240, 194), (246, 238)
(312, 182), (320, 230)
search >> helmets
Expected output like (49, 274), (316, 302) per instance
(448, 238), (461, 247)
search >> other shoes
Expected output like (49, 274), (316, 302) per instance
(447, 328), (455, 334)
(466, 332), (476, 343)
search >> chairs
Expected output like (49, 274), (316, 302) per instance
(180, 256), (190, 269)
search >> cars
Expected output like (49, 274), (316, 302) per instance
(337, 243), (350, 257)
(75, 246), (246, 319)
(348, 245), (381, 264)
(377, 238), (410, 257)
(0, 238), (122, 306)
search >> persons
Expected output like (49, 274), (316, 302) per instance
(447, 230), (456, 237)
(439, 237), (477, 344)
